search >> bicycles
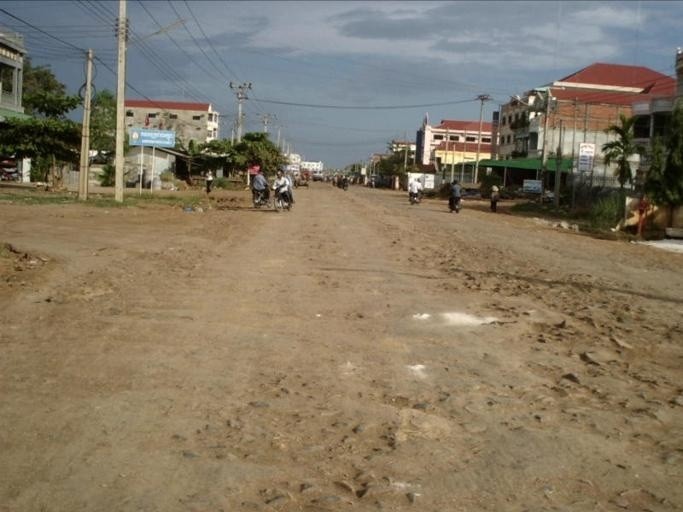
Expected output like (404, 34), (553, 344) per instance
(274, 187), (294, 212)
(251, 182), (273, 209)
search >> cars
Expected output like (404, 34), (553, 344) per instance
(294, 173), (310, 189)
(313, 170), (326, 182)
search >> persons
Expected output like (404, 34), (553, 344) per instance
(252, 170), (270, 201)
(205, 168), (213, 192)
(488, 182), (500, 212)
(448, 180), (461, 204)
(409, 178), (424, 201)
(272, 168), (294, 208)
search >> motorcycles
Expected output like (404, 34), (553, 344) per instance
(448, 193), (462, 214)
(408, 191), (420, 206)
(328, 175), (349, 192)
